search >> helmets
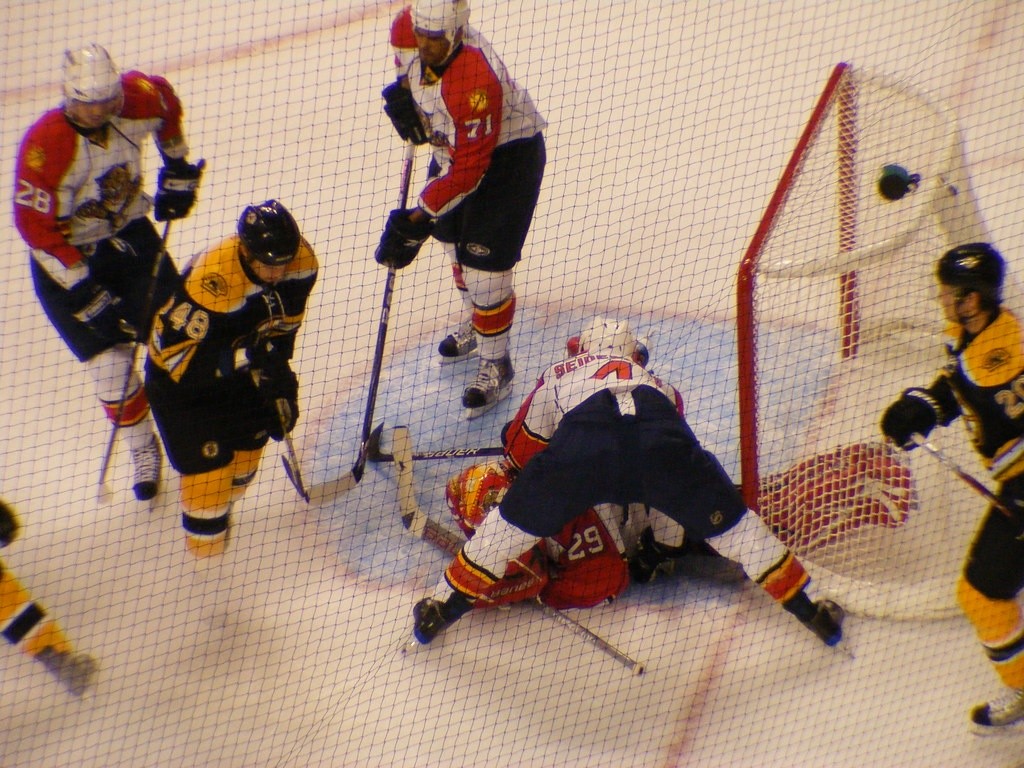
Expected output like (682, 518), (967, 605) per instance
(61, 42), (125, 117)
(237, 199), (301, 265)
(410, 0), (470, 65)
(935, 242), (1006, 310)
(565, 315), (649, 366)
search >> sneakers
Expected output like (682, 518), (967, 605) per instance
(809, 597), (848, 655)
(462, 352), (517, 420)
(407, 595), (451, 648)
(128, 431), (164, 512)
(969, 688), (1024, 735)
(63, 649), (100, 705)
(438, 313), (477, 364)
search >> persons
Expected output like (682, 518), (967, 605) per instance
(12, 42), (202, 502)
(411, 315), (845, 645)
(878, 242), (1024, 728)
(369, 0), (549, 409)
(141, 199), (322, 578)
(0, 497), (97, 697)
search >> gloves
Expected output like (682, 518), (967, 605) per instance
(67, 276), (135, 342)
(235, 370), (299, 441)
(882, 374), (963, 450)
(153, 158), (206, 222)
(382, 81), (433, 144)
(376, 208), (435, 269)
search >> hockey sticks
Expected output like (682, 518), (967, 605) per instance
(367, 422), (507, 463)
(96, 219), (171, 506)
(265, 339), (305, 499)
(911, 430), (1024, 531)
(390, 424), (646, 677)
(306, 136), (415, 506)
